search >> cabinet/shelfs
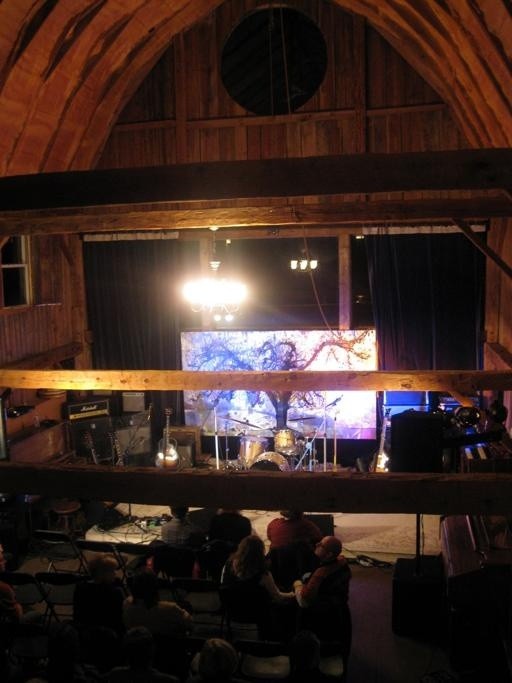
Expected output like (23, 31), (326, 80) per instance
(2, 341), (84, 464)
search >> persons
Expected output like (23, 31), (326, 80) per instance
(2, 505), (351, 682)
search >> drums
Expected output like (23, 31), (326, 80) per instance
(238, 434), (270, 467)
(273, 429), (301, 456)
(250, 451), (290, 472)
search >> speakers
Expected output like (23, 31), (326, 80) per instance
(390, 555), (448, 637)
(390, 408), (443, 472)
(64, 397), (109, 420)
(64, 416), (116, 463)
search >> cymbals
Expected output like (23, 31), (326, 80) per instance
(232, 418), (262, 430)
(217, 415), (234, 421)
(288, 415), (317, 422)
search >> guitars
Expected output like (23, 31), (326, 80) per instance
(155, 408), (180, 468)
(367, 409), (390, 473)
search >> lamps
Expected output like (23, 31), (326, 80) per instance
(289, 243), (318, 272)
(180, 229), (247, 315)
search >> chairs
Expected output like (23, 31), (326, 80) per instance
(1, 528), (347, 683)
